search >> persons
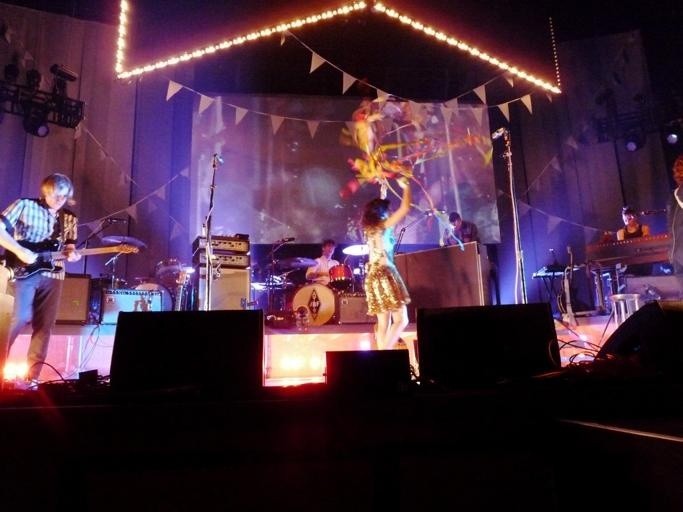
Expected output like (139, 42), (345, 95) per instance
(0, 173), (82, 383)
(671, 156), (683, 301)
(444, 213), (480, 246)
(617, 208), (653, 278)
(362, 177), (411, 349)
(306, 239), (337, 285)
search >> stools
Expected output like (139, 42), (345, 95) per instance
(610, 290), (639, 330)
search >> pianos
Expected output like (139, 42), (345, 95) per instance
(584, 233), (673, 266)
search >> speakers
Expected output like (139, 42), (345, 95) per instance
(417, 303), (561, 383)
(593, 301), (683, 361)
(109, 309), (265, 386)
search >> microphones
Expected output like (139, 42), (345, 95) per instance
(641, 208), (665, 216)
(282, 238), (295, 242)
(106, 217), (128, 224)
(491, 127), (506, 140)
(215, 154), (224, 165)
(427, 209), (445, 216)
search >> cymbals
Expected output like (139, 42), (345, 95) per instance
(278, 257), (318, 268)
(351, 268), (368, 274)
(102, 235), (147, 250)
(342, 244), (368, 256)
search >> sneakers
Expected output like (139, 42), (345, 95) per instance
(24, 378), (39, 391)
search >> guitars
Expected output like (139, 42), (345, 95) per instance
(556, 246), (580, 314)
(5, 238), (139, 280)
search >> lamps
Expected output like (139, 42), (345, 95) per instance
(624, 119), (681, 153)
(0, 61), (83, 139)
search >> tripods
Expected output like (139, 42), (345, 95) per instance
(262, 242), (296, 323)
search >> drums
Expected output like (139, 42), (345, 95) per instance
(123, 277), (175, 312)
(287, 282), (336, 326)
(329, 263), (354, 291)
(154, 257), (182, 280)
